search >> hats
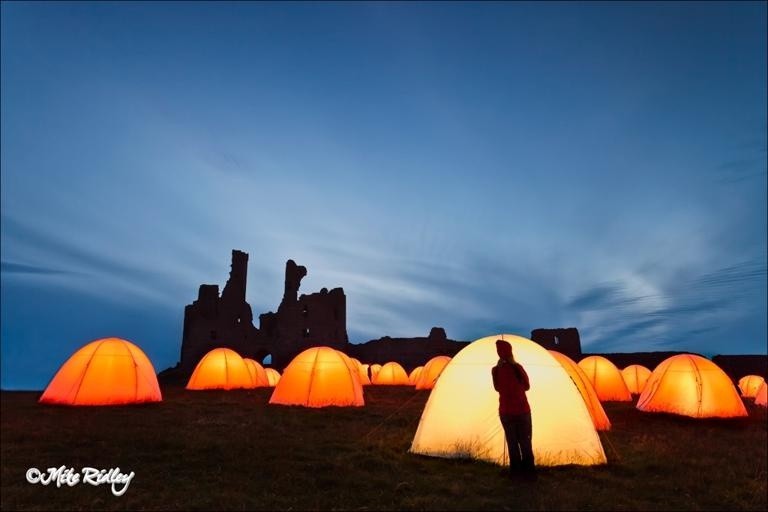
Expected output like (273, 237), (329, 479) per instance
(496, 340), (512, 358)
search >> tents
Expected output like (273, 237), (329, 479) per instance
(411, 334), (608, 467)
(242, 352), (768, 420)
(38, 338), (165, 405)
(186, 348), (256, 391)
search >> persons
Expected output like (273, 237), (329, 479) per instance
(493, 341), (535, 467)
